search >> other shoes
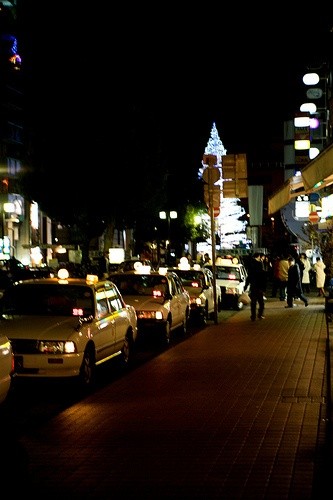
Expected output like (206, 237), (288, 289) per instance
(318, 295), (321, 296)
(285, 305), (293, 308)
(305, 302), (308, 306)
(260, 316), (265, 319)
(323, 296), (326, 297)
(281, 298), (284, 301)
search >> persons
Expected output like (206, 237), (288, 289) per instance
(98, 250), (110, 281)
(204, 253), (211, 265)
(185, 271), (203, 286)
(216, 252), (327, 323)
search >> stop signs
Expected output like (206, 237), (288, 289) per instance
(208, 207), (220, 218)
(309, 212), (320, 223)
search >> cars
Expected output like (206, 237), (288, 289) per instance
(108, 272), (192, 346)
(167, 268), (222, 329)
(117, 260), (157, 275)
(202, 263), (252, 311)
(0, 259), (55, 282)
(0, 332), (15, 404)
(0, 279), (138, 391)
